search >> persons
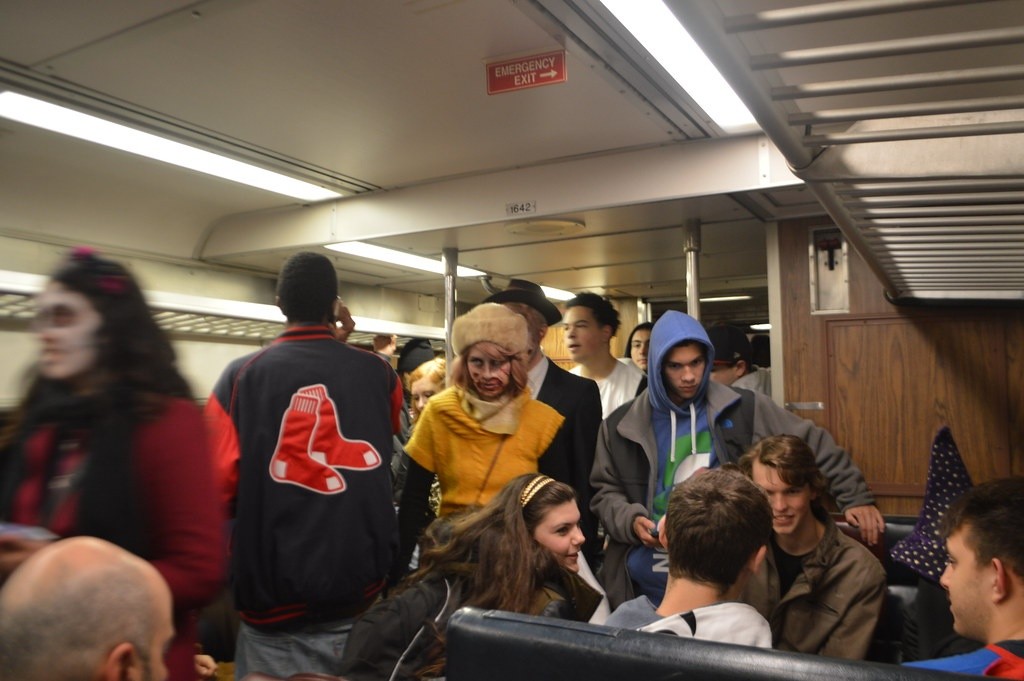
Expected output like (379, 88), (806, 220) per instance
(0, 249), (229, 681)
(738, 436), (887, 661)
(206, 251), (401, 681)
(706, 324), (771, 397)
(899, 476), (1024, 681)
(0, 536), (176, 681)
(196, 602), (236, 681)
(374, 279), (603, 589)
(606, 469), (772, 649)
(590, 309), (885, 613)
(563, 293), (647, 419)
(342, 473), (603, 681)
(625, 323), (654, 371)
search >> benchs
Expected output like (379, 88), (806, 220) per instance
(445, 518), (1024, 681)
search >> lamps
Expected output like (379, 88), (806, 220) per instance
(1, 83), (356, 202)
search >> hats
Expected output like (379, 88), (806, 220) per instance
(705, 325), (750, 363)
(451, 302), (526, 354)
(482, 281), (562, 326)
(396, 338), (434, 373)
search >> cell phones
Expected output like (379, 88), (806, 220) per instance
(333, 296), (342, 321)
